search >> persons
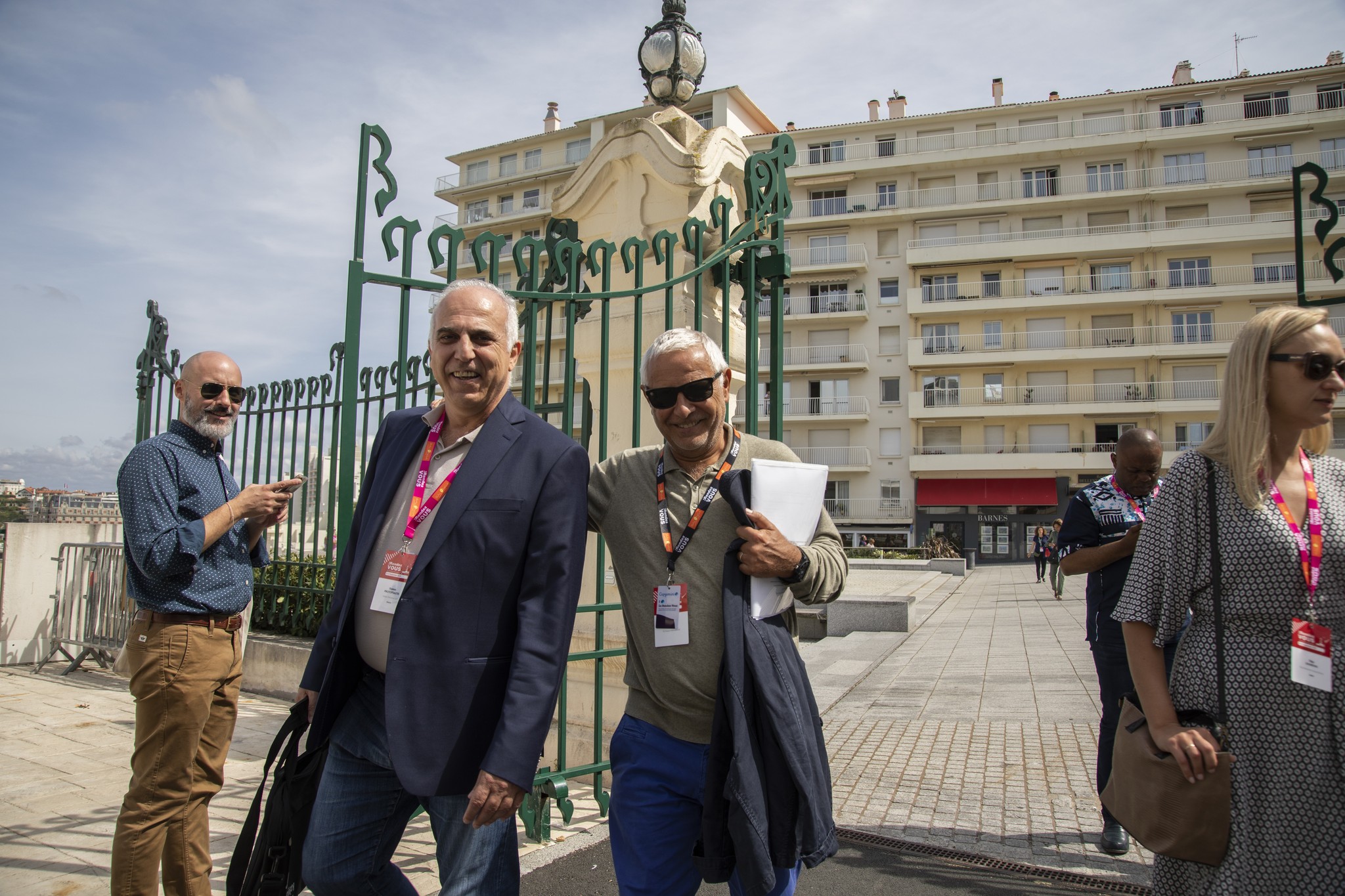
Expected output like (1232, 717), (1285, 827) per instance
(1027, 525), (1049, 584)
(110, 349), (305, 896)
(586, 326), (850, 896)
(1109, 304), (1345, 896)
(1044, 519), (1065, 600)
(296, 281), (591, 896)
(1057, 428), (1193, 852)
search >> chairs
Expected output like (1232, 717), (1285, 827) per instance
(839, 300), (850, 312)
(1104, 337), (1116, 347)
(1126, 283), (1141, 292)
(784, 304), (790, 315)
(1082, 288), (1102, 294)
(923, 450), (942, 453)
(1030, 290), (1042, 297)
(1063, 288), (1076, 295)
(741, 306), (746, 318)
(870, 202), (880, 211)
(826, 302), (835, 313)
(1125, 336), (1136, 347)
(953, 345), (965, 354)
(845, 206), (854, 213)
(925, 347), (936, 355)
(827, 504), (838, 517)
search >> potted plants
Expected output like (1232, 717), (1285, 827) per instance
(1022, 388), (1035, 405)
(837, 503), (846, 515)
(1123, 385), (1142, 402)
(839, 355), (846, 362)
(854, 289), (863, 311)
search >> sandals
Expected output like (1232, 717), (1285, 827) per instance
(1057, 594), (1062, 600)
(1055, 590), (1058, 598)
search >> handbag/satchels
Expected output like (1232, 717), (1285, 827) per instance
(1099, 684), (1233, 870)
(1047, 545), (1060, 564)
(225, 696), (331, 896)
(1045, 548), (1050, 557)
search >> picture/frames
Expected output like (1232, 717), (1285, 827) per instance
(1026, 526), (1054, 554)
(981, 526), (1009, 554)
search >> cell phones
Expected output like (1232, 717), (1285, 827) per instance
(275, 474), (308, 494)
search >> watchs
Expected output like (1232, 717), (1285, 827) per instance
(777, 546), (810, 584)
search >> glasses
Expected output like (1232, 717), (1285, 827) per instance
(182, 379), (248, 404)
(1268, 351), (1345, 382)
(644, 368), (722, 409)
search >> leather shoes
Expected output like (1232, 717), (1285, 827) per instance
(1100, 820), (1130, 854)
(1036, 580), (1041, 583)
(1041, 576), (1046, 582)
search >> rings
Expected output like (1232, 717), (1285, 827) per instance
(740, 553), (744, 563)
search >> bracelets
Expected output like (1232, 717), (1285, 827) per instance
(225, 502), (234, 529)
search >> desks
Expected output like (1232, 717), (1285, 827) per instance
(1112, 339), (1126, 347)
(830, 302), (844, 312)
(1044, 287), (1059, 296)
(1110, 286), (1122, 292)
(921, 452), (946, 454)
(852, 204), (866, 212)
(935, 348), (949, 354)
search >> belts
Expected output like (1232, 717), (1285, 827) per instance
(136, 608), (243, 632)
